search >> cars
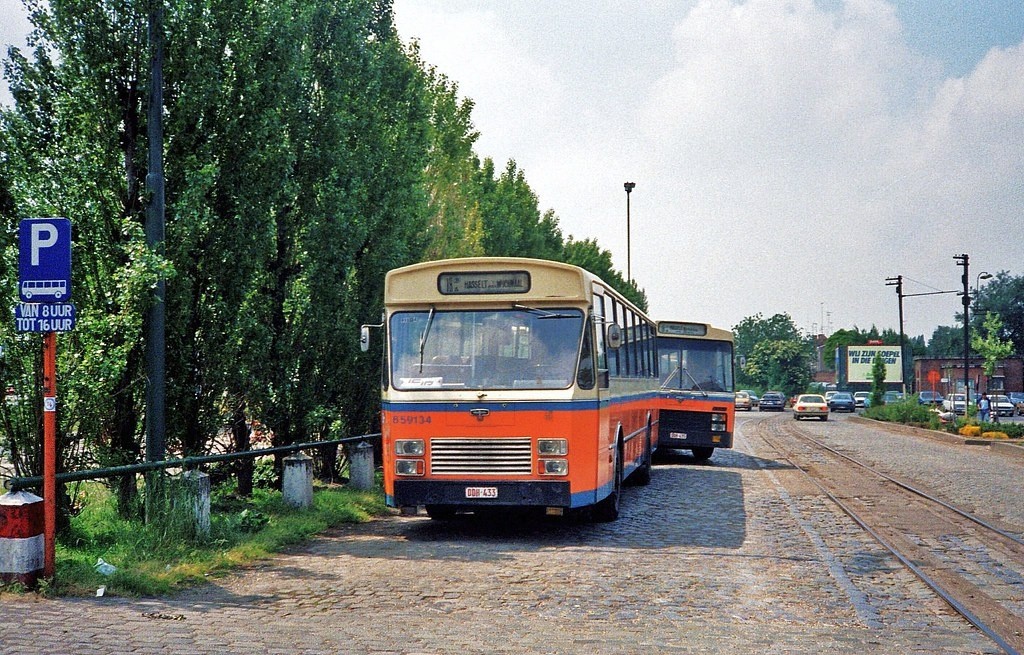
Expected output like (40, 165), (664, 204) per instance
(881, 390), (908, 405)
(972, 394), (982, 404)
(1006, 391), (1024, 405)
(735, 392), (752, 411)
(1015, 401), (1024, 416)
(942, 394), (974, 414)
(918, 391), (945, 406)
(792, 394), (830, 421)
(853, 391), (870, 407)
(740, 389), (759, 407)
(765, 390), (787, 405)
(825, 390), (839, 407)
(830, 392), (857, 413)
(758, 393), (785, 412)
(986, 394), (1015, 417)
(790, 395), (798, 408)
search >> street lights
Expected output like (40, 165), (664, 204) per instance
(624, 182), (637, 283)
(963, 270), (996, 419)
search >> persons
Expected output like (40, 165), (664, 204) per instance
(976, 393), (990, 423)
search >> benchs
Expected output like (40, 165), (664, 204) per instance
(430, 355), (531, 387)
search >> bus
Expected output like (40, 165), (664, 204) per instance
(360, 256), (663, 523)
(608, 320), (745, 462)
